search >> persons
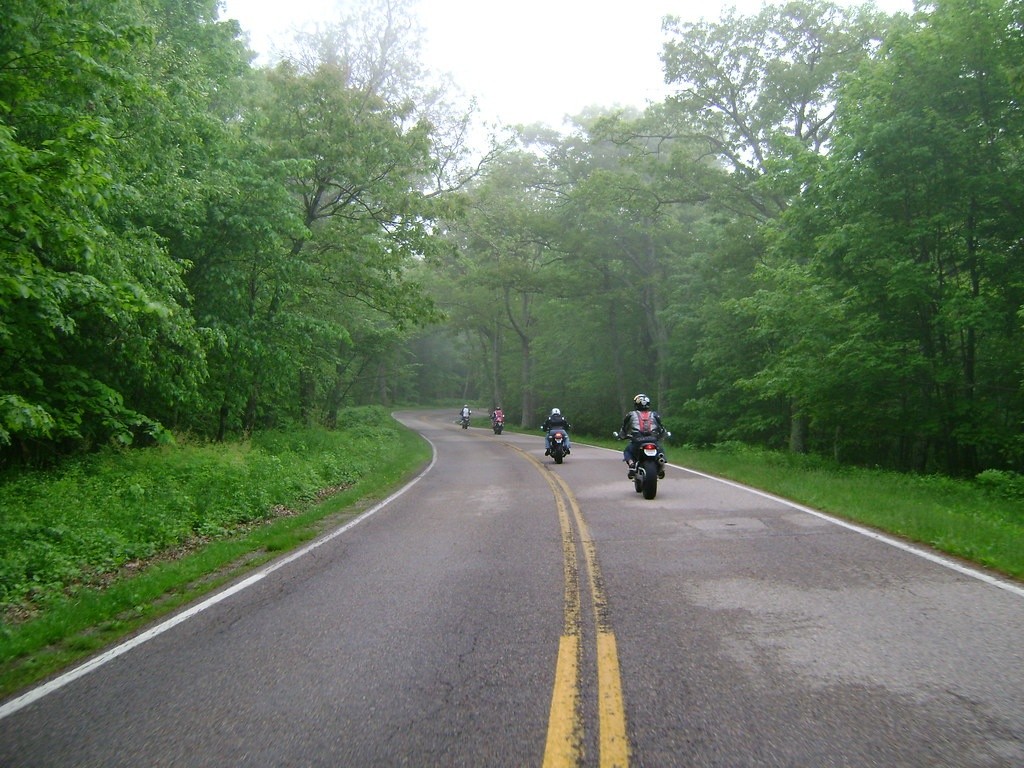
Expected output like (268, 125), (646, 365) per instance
(542, 408), (571, 456)
(617, 394), (667, 479)
(492, 407), (505, 427)
(460, 404), (472, 425)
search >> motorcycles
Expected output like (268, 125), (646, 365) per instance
(612, 429), (672, 500)
(489, 414), (505, 434)
(460, 409), (471, 429)
(540, 424), (571, 464)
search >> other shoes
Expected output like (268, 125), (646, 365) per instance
(658, 470), (665, 479)
(628, 463), (635, 479)
(567, 449), (570, 454)
(545, 451), (549, 456)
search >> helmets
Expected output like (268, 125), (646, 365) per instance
(464, 404), (468, 408)
(634, 393), (651, 410)
(496, 407), (501, 410)
(552, 408), (560, 415)
